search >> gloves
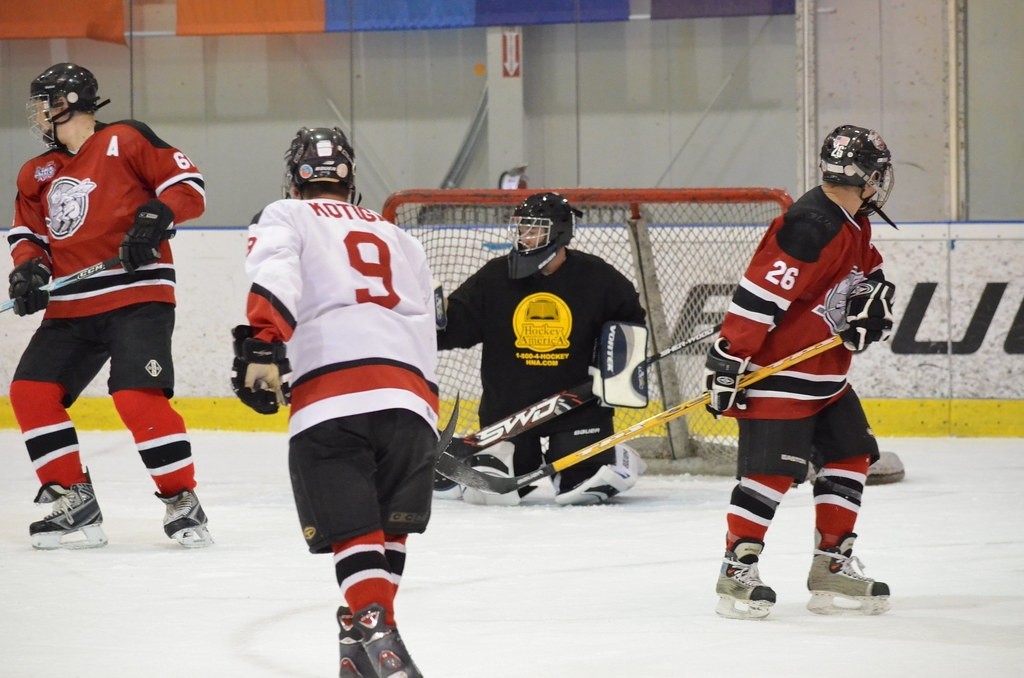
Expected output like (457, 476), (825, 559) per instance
(119, 199), (177, 274)
(230, 325), (292, 415)
(702, 336), (752, 420)
(840, 278), (896, 354)
(9, 257), (53, 317)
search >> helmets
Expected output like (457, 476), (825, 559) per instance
(819, 125), (899, 231)
(505, 191), (584, 280)
(25, 63), (110, 149)
(281, 126), (362, 206)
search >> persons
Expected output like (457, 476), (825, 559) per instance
(433, 193), (649, 504)
(704, 124), (896, 620)
(6, 63), (215, 550)
(229, 126), (446, 678)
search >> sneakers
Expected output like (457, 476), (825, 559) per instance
(458, 440), (521, 507)
(807, 528), (890, 615)
(716, 531), (776, 620)
(29, 465), (108, 549)
(543, 450), (637, 505)
(337, 603), (422, 678)
(154, 487), (217, 548)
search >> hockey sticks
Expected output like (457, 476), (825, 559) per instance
(1, 253), (121, 314)
(437, 390), (459, 467)
(433, 320), (721, 459)
(436, 325), (861, 495)
